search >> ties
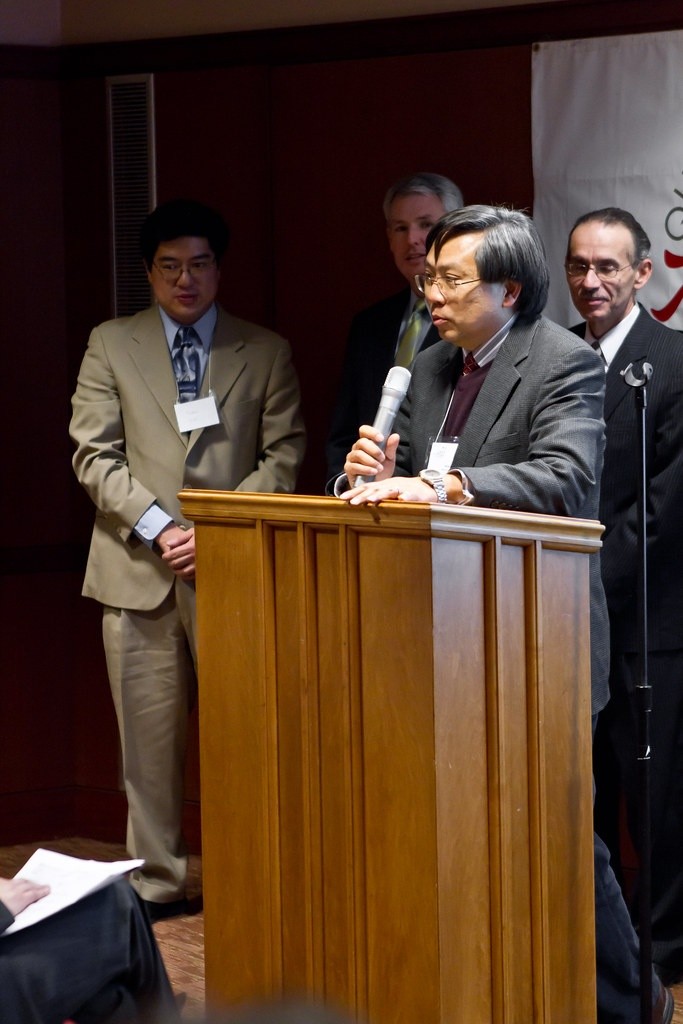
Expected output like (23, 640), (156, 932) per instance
(171, 326), (200, 403)
(590, 341), (603, 360)
(393, 299), (426, 368)
(461, 352), (480, 375)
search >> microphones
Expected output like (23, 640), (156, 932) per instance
(352, 366), (411, 489)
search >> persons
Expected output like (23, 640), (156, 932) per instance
(563, 208), (683, 990)
(325, 204), (673, 1024)
(0, 877), (180, 1024)
(324, 173), (465, 497)
(69, 200), (307, 920)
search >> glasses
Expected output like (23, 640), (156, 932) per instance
(146, 251), (220, 280)
(563, 261), (644, 278)
(415, 274), (511, 297)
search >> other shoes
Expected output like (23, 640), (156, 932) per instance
(654, 969), (683, 986)
(141, 890), (188, 924)
(648, 980), (675, 1024)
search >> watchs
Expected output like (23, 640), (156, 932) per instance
(419, 469), (447, 504)
